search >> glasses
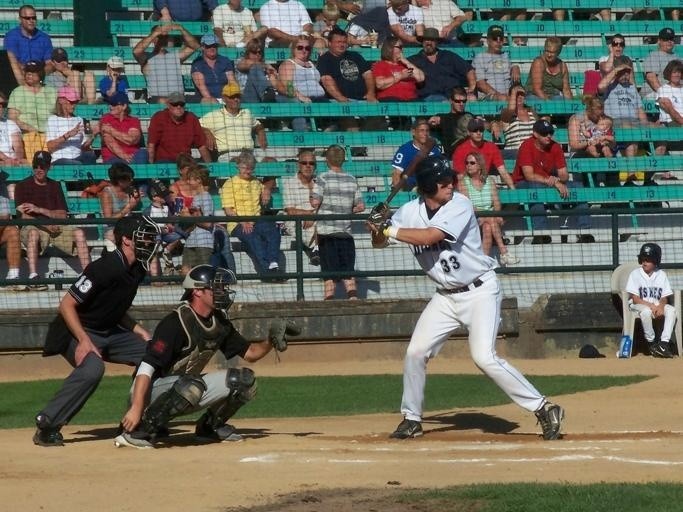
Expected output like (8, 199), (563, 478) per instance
(170, 102), (184, 106)
(299, 160), (315, 166)
(294, 45), (311, 51)
(20, 15), (36, 20)
(662, 37), (673, 41)
(611, 41), (624, 47)
(249, 50), (263, 55)
(452, 98), (468, 103)
(464, 161), (479, 165)
(393, 45), (403, 50)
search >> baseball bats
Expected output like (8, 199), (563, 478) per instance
(385, 140), (435, 204)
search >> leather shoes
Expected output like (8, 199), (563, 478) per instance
(535, 401), (564, 440)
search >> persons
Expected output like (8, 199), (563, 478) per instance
(1, 1), (682, 302)
(31, 210), (164, 447)
(363, 153), (568, 441)
(114, 263), (303, 449)
(623, 243), (678, 359)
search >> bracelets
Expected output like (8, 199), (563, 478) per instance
(387, 225), (400, 239)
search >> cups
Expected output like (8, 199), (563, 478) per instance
(173, 196), (184, 213)
(276, 221), (285, 235)
(560, 226), (568, 243)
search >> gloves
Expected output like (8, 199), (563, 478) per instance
(368, 207), (392, 234)
(366, 205), (387, 236)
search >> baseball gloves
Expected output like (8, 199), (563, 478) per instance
(268, 318), (301, 353)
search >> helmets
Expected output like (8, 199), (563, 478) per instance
(638, 243), (661, 267)
(415, 157), (456, 185)
(180, 264), (237, 311)
(114, 215), (162, 272)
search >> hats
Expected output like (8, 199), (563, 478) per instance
(147, 180), (174, 197)
(221, 83), (241, 97)
(487, 25), (504, 38)
(417, 28), (444, 45)
(468, 118), (485, 130)
(21, 48), (124, 101)
(33, 151), (51, 166)
(166, 92), (184, 103)
(579, 344), (606, 358)
(201, 34), (220, 46)
(533, 119), (553, 134)
(659, 28), (674, 39)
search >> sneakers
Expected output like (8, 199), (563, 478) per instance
(656, 341), (673, 358)
(5, 277), (30, 291)
(647, 342), (662, 358)
(194, 427), (244, 443)
(499, 251), (520, 265)
(310, 250), (320, 265)
(113, 424), (169, 449)
(29, 275), (48, 291)
(390, 415), (423, 441)
(33, 428), (65, 447)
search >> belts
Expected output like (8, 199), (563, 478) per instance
(436, 278), (482, 294)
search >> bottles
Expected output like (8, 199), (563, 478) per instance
(286, 80), (294, 98)
(47, 268), (64, 291)
(618, 335), (632, 359)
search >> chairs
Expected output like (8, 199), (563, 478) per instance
(611, 263), (683, 358)
(0, 0), (683, 246)
